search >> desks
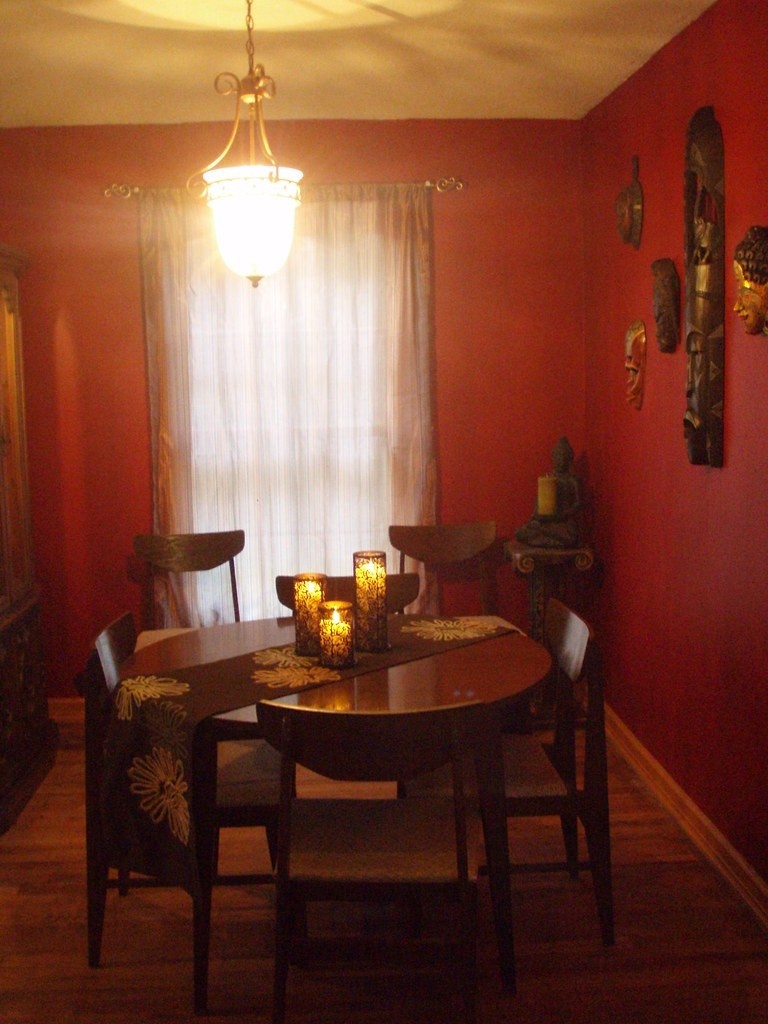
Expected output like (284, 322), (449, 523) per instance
(121, 623), (551, 1018)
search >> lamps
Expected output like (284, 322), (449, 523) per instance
(185, 0), (304, 291)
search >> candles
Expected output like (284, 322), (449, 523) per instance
(293, 574), (330, 654)
(537, 472), (559, 516)
(354, 549), (388, 652)
(318, 600), (355, 668)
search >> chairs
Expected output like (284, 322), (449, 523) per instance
(387, 522), (497, 617)
(274, 572), (421, 785)
(131, 528), (245, 627)
(253, 698), (487, 1024)
(396, 597), (613, 948)
(77, 609), (289, 968)
